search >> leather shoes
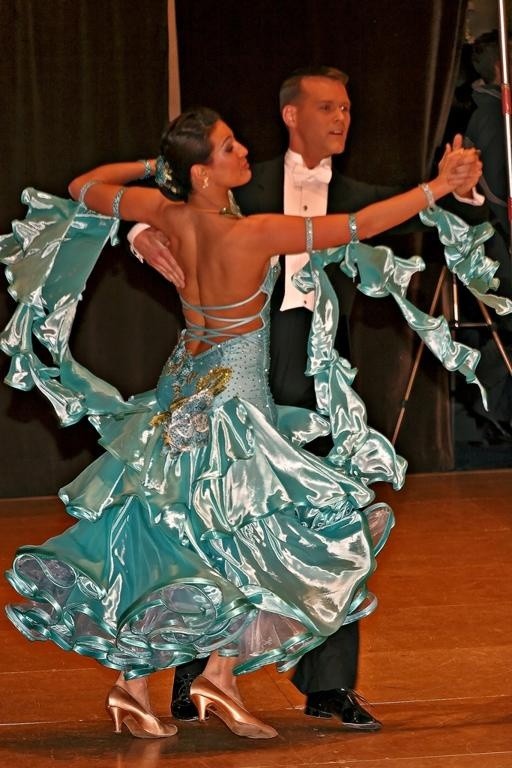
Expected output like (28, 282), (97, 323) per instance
(171, 656), (210, 721)
(304, 687), (383, 730)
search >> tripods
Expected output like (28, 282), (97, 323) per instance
(393, 253), (512, 459)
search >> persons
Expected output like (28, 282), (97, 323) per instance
(464, 30), (512, 294)
(0, 111), (472, 739)
(113, 67), (483, 729)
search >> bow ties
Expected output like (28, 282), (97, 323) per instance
(288, 159), (338, 188)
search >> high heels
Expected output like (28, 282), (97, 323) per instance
(188, 672), (279, 740)
(105, 685), (178, 739)
